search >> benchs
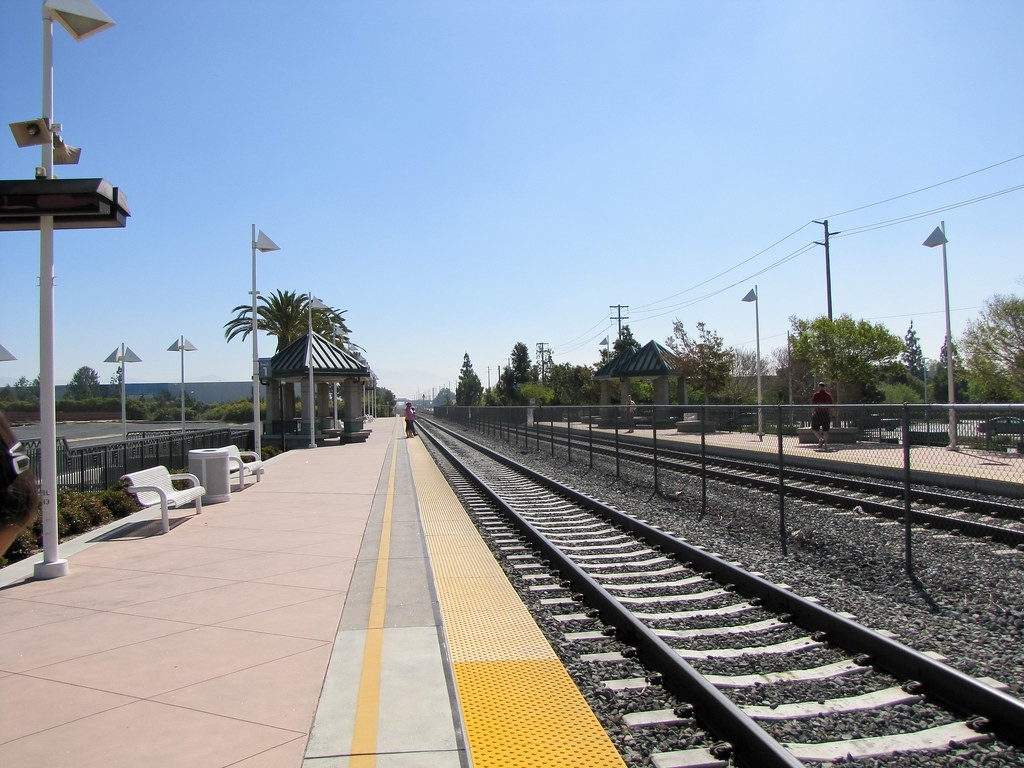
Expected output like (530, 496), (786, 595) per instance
(120, 465), (206, 533)
(221, 445), (264, 489)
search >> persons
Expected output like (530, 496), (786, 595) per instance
(405, 402), (418, 438)
(624, 394), (638, 432)
(0, 409), (38, 561)
(810, 381), (837, 451)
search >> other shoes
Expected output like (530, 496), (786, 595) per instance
(818, 439), (824, 448)
(414, 434), (419, 436)
(823, 448), (828, 452)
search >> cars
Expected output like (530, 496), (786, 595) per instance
(977, 416), (1024, 436)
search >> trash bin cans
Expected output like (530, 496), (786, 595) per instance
(188, 448), (231, 503)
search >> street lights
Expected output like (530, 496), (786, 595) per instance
(741, 284), (765, 436)
(307, 290), (329, 449)
(250, 222), (280, 475)
(923, 219), (961, 452)
(350, 343), (380, 421)
(103, 341), (143, 440)
(166, 333), (198, 433)
(331, 321), (346, 430)
